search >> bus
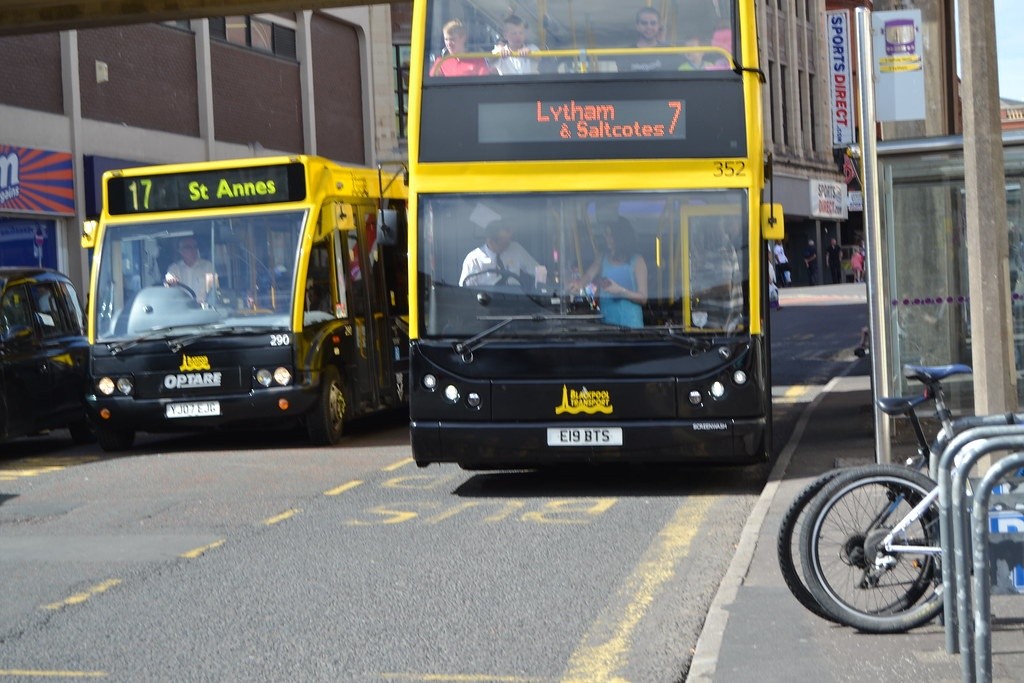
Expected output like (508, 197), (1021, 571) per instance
(82, 152), (413, 447)
(403, 0), (787, 469)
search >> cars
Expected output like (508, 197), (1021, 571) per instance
(0, 266), (88, 448)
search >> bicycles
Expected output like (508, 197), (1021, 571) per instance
(776, 397), (1024, 625)
(799, 365), (1023, 633)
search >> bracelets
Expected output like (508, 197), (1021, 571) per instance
(621, 287), (625, 295)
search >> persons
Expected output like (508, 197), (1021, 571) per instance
(851, 245), (864, 283)
(678, 36), (713, 71)
(687, 212), (743, 334)
(164, 237), (221, 312)
(768, 245), (783, 311)
(457, 221), (561, 298)
(34, 229), (44, 270)
(774, 237), (791, 287)
(568, 214), (648, 329)
(616, 7), (687, 72)
(826, 239), (844, 285)
(491, 15), (542, 75)
(804, 240), (817, 285)
(428, 18), (490, 77)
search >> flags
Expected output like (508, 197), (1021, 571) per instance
(841, 155), (857, 184)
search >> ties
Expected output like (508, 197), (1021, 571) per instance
(495, 252), (505, 270)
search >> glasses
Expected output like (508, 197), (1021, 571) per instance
(638, 19), (659, 26)
